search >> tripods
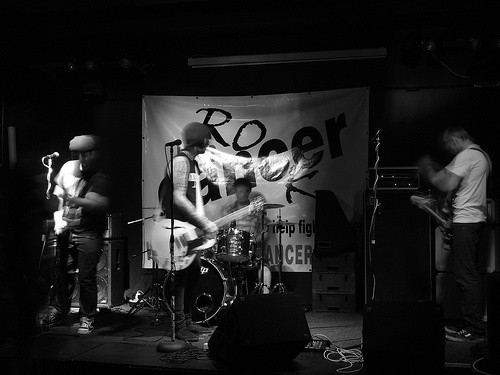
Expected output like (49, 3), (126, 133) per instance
(252, 210), (289, 294)
(126, 215), (174, 326)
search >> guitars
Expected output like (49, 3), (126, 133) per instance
(150, 195), (267, 272)
(412, 195), (452, 245)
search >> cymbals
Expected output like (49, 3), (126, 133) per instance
(266, 222), (298, 226)
(264, 204), (285, 209)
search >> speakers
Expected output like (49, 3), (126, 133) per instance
(67, 238), (125, 307)
(363, 189), (434, 304)
(208, 294), (314, 368)
(361, 313), (446, 371)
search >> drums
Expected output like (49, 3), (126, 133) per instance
(215, 228), (251, 263)
(162, 258), (238, 323)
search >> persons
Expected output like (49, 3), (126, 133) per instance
(419, 123), (491, 343)
(151, 122), (272, 341)
(36, 134), (122, 334)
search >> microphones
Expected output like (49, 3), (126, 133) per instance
(164, 138), (183, 148)
(41, 151), (59, 161)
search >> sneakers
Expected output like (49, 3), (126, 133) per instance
(445, 325), (485, 343)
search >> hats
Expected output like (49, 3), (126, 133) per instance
(181, 122), (212, 148)
(68, 135), (94, 151)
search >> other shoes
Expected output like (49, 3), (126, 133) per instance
(77, 321), (95, 334)
(177, 328), (199, 341)
(187, 324), (203, 331)
(39, 314), (66, 325)
